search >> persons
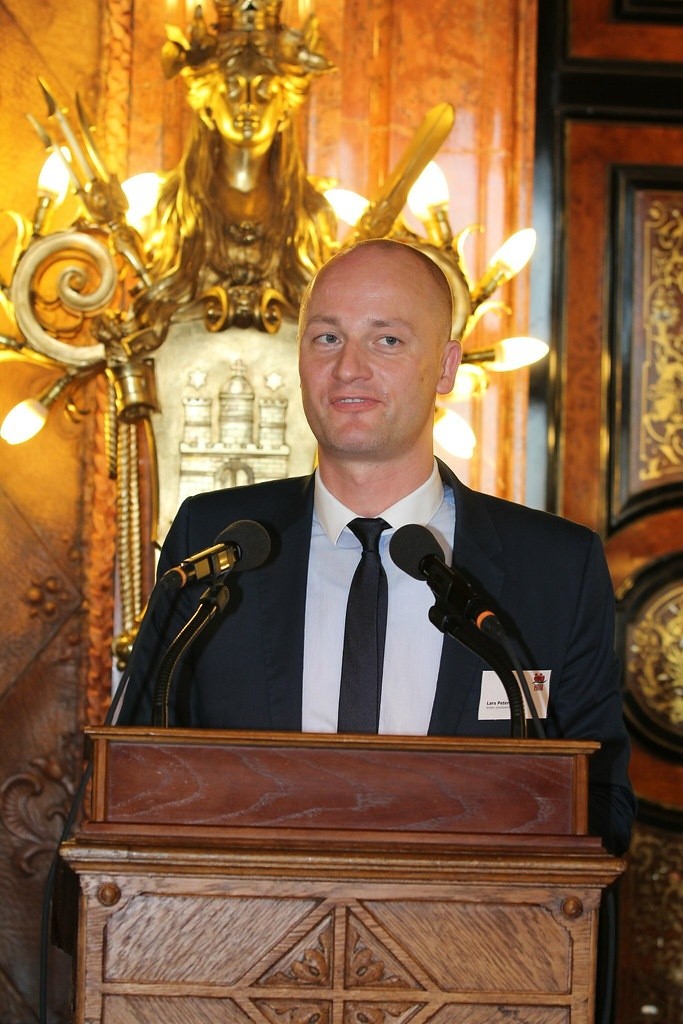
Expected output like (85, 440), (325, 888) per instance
(136, 0), (338, 302)
(105, 240), (637, 861)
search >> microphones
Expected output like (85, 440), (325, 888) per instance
(389, 525), (504, 638)
(156, 520), (273, 592)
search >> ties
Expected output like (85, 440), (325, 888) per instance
(337, 517), (392, 734)
